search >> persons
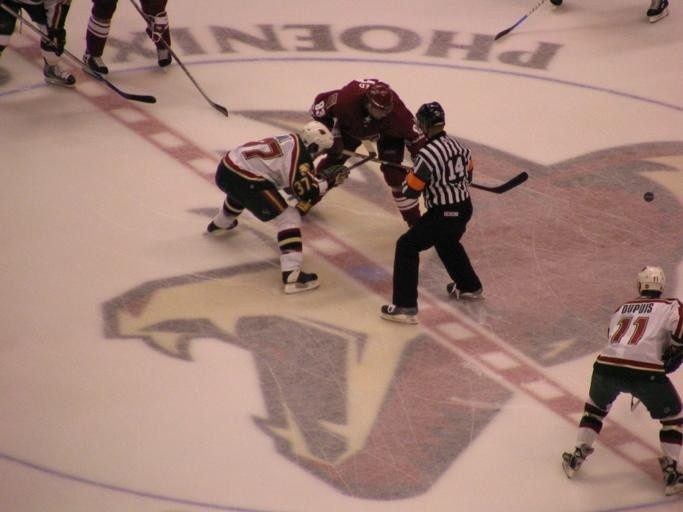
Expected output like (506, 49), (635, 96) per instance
(549, 0), (669, 15)
(82, 0), (176, 75)
(299, 78), (423, 227)
(562, 265), (683, 487)
(207, 121), (336, 282)
(382, 102), (482, 317)
(0, 0), (77, 87)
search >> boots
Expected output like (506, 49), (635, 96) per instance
(563, 444), (594, 470)
(658, 457), (683, 485)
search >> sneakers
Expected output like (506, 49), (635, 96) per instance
(44, 64), (75, 84)
(447, 283), (482, 297)
(647, 0), (668, 16)
(382, 304), (417, 315)
(157, 48), (171, 66)
(282, 270), (317, 283)
(208, 219), (237, 231)
(83, 53), (108, 72)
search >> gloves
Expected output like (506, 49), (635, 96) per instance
(41, 29), (65, 55)
(321, 165), (350, 188)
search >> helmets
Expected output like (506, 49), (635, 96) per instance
(417, 101), (444, 133)
(637, 265), (664, 293)
(366, 83), (393, 111)
(301, 121), (334, 154)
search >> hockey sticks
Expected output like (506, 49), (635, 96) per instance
(130, 0), (228, 117)
(342, 149), (528, 193)
(494, 0), (547, 42)
(0, 3), (156, 103)
(348, 140), (377, 169)
